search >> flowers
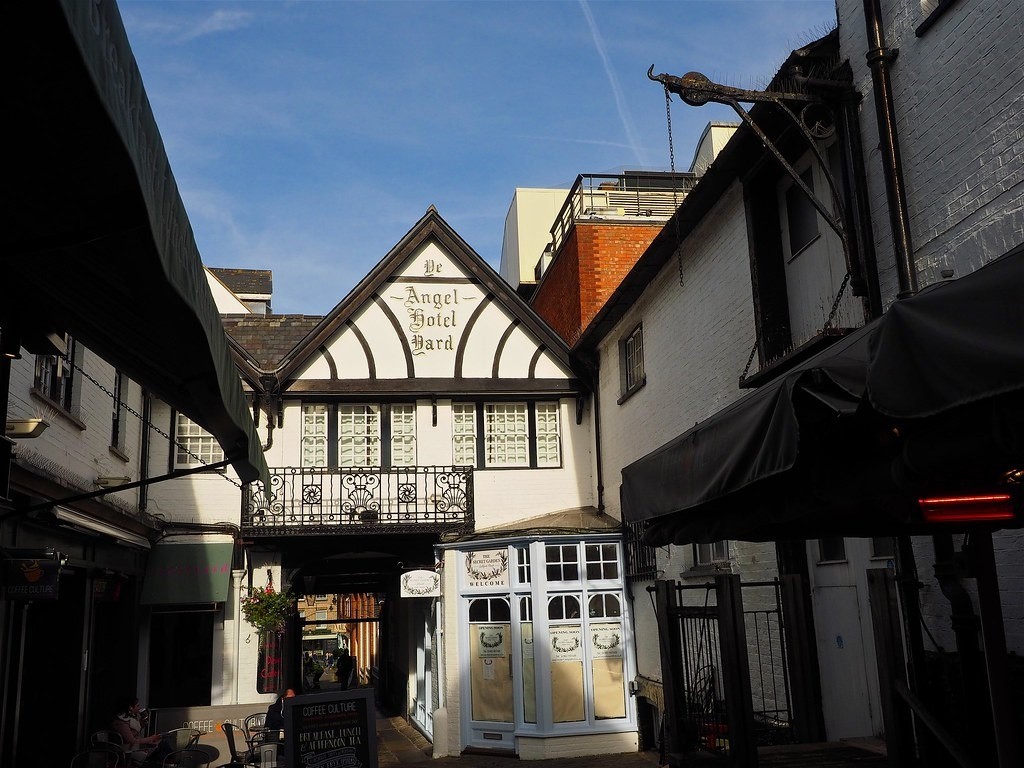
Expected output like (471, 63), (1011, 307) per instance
(241, 588), (295, 650)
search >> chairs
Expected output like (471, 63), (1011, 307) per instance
(71, 749), (119, 768)
(657, 667), (716, 766)
(147, 729), (206, 768)
(244, 712), (268, 749)
(221, 723), (254, 766)
(93, 741), (131, 768)
(91, 730), (123, 750)
(248, 731), (286, 768)
(163, 750), (210, 768)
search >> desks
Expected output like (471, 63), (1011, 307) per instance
(118, 743), (158, 768)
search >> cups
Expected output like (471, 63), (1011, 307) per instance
(139, 709), (148, 720)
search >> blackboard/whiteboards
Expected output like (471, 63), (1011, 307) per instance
(283, 686), (380, 768)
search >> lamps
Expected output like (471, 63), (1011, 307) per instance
(5, 418), (49, 438)
(361, 510), (378, 524)
(94, 475), (132, 488)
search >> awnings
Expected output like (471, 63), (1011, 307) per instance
(0, 0), (271, 494)
(141, 534), (235, 602)
(621, 241), (1024, 547)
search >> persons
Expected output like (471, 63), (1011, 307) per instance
(336, 647), (352, 691)
(303, 653), (313, 677)
(262, 689), (295, 757)
(303, 658), (324, 689)
(110, 695), (160, 768)
